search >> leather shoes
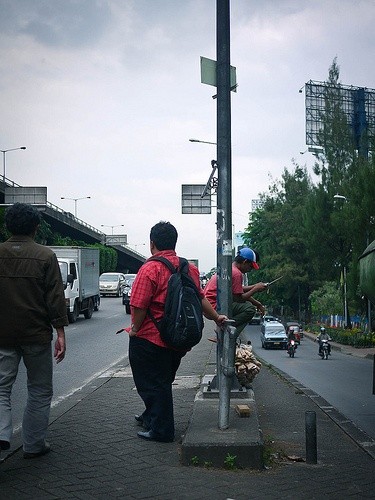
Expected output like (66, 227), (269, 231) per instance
(138, 431), (175, 444)
(134, 414), (150, 429)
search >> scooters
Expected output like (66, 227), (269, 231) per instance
(316, 336), (333, 360)
(300, 329), (303, 337)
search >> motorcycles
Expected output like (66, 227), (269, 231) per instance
(286, 339), (297, 358)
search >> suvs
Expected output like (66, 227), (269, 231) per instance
(260, 319), (288, 349)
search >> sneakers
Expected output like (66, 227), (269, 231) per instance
(0, 450), (8, 461)
(23, 442), (50, 458)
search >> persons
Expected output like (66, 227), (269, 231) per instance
(316, 327), (332, 356)
(0, 202), (69, 459)
(286, 328), (297, 349)
(201, 248), (268, 344)
(115, 222), (226, 443)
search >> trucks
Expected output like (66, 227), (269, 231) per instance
(45, 245), (101, 324)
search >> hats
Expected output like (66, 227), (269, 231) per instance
(238, 247), (259, 269)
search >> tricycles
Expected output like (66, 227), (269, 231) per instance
(289, 326), (301, 344)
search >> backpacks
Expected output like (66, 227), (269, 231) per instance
(146, 256), (203, 352)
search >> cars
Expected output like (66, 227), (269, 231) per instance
(250, 309), (272, 325)
(99, 273), (125, 297)
(124, 274), (137, 314)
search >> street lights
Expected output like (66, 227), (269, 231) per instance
(61, 196), (91, 216)
(135, 244), (146, 246)
(0, 146), (26, 181)
(100, 224), (124, 235)
